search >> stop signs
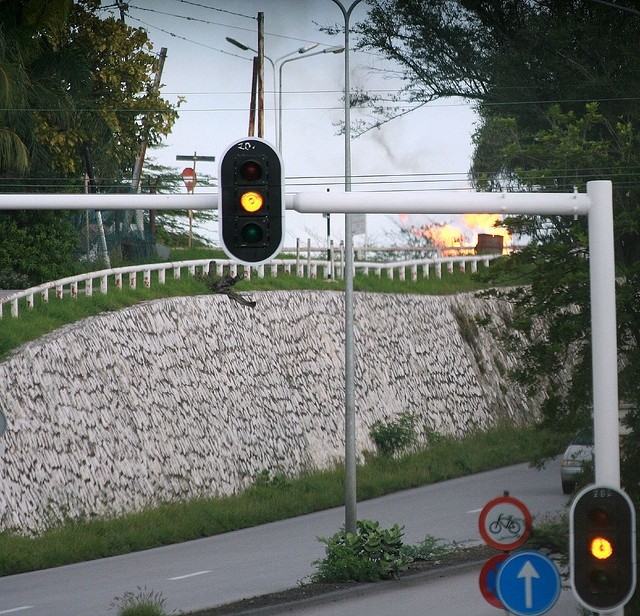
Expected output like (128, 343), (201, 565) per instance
(180, 169), (198, 190)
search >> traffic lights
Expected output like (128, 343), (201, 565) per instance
(568, 483), (636, 611)
(218, 136), (286, 266)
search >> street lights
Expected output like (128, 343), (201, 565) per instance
(226, 35), (320, 150)
(279, 47), (345, 172)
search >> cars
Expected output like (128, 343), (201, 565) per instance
(562, 403), (640, 491)
